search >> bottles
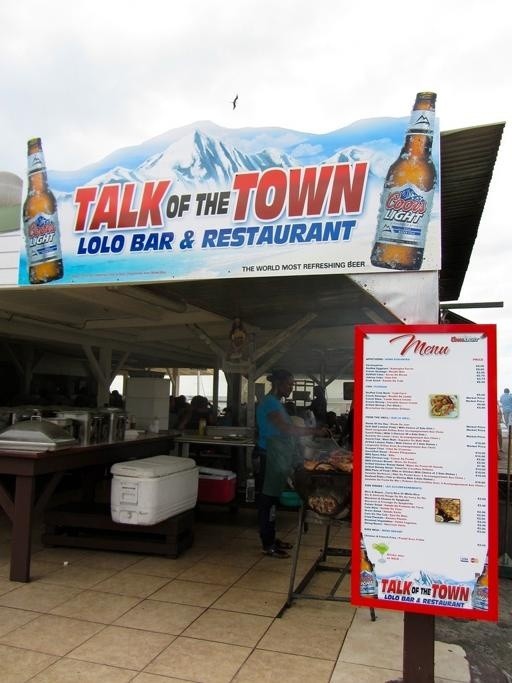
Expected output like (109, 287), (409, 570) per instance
(368, 90), (437, 270)
(358, 531), (378, 598)
(199, 417), (207, 435)
(130, 414), (136, 429)
(22, 134), (65, 285)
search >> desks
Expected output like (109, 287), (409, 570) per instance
(173, 437), (256, 503)
(0, 430), (189, 582)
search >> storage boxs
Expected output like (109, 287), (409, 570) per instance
(110, 455), (199, 526)
(196, 465), (236, 504)
(125, 377), (170, 431)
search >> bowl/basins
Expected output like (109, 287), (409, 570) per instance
(280, 491), (303, 506)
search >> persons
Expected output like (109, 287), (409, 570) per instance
(1, 383), (352, 445)
(499, 387), (512, 426)
(248, 365), (334, 559)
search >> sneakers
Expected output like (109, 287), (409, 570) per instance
(274, 537), (293, 549)
(261, 544), (290, 558)
